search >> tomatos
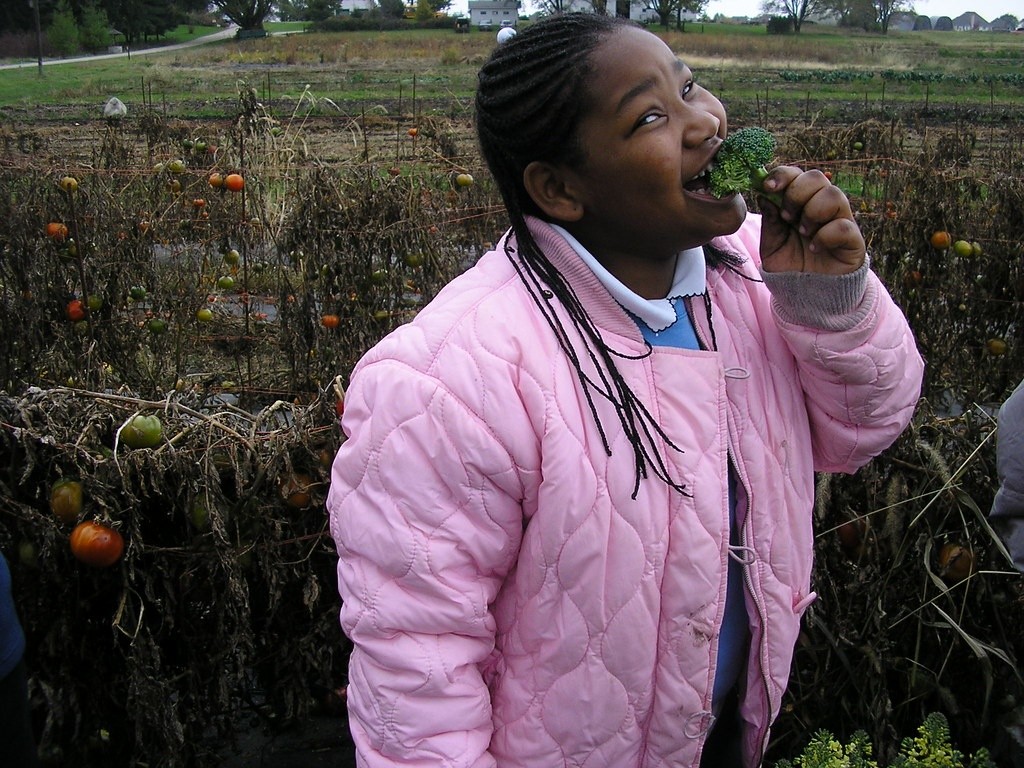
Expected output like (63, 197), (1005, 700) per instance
(900, 230), (1005, 354)
(44, 139), (474, 565)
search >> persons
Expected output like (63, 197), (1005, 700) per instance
(349, 12), (925, 768)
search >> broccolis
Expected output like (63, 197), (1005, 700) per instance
(709, 126), (785, 207)
